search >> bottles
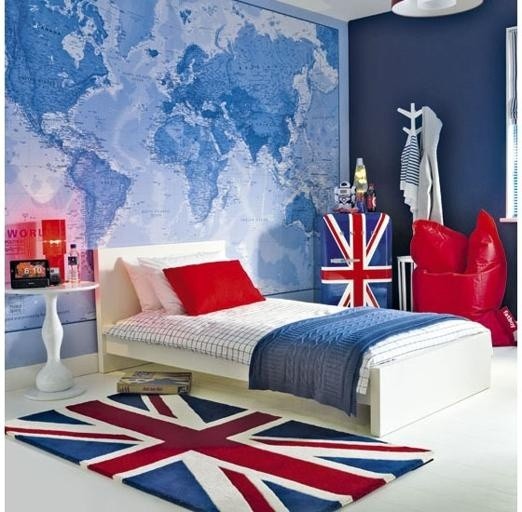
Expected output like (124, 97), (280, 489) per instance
(68, 244), (79, 286)
(354, 157), (377, 212)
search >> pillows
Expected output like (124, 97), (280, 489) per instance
(119, 251), (266, 318)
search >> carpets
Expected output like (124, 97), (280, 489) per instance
(5, 393), (433, 511)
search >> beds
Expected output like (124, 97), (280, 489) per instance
(93, 239), (492, 437)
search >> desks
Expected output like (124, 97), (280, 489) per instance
(6, 280), (101, 400)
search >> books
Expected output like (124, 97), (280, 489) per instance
(116, 369), (192, 394)
